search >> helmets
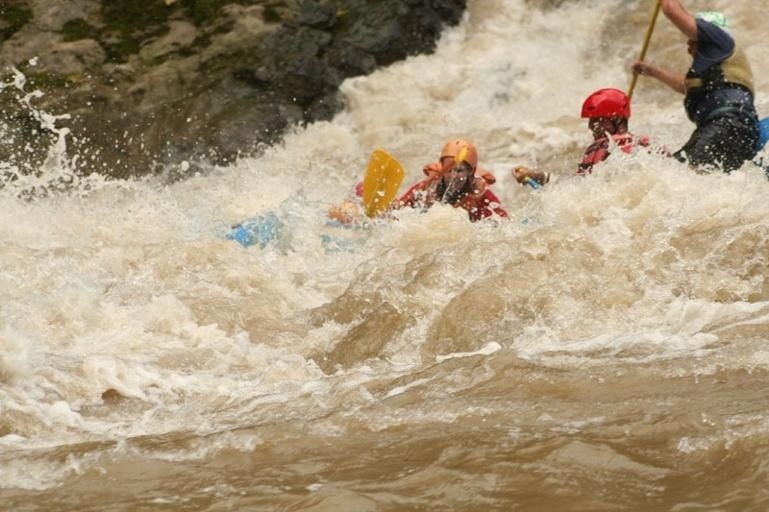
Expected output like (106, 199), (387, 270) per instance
(698, 12), (730, 33)
(440, 140), (477, 170)
(581, 88), (631, 119)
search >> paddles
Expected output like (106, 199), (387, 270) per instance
(363, 149), (405, 219)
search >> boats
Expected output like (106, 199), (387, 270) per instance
(220, 116), (768, 257)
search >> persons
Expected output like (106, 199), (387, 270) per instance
(630, 0), (762, 176)
(326, 138), (510, 226)
(511, 88), (650, 187)
